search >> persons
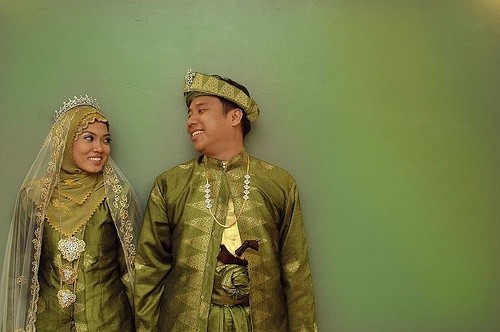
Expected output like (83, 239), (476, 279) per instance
(0, 92), (146, 332)
(135, 66), (319, 332)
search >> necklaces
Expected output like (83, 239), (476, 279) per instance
(56, 170), (102, 310)
(201, 152), (252, 229)
